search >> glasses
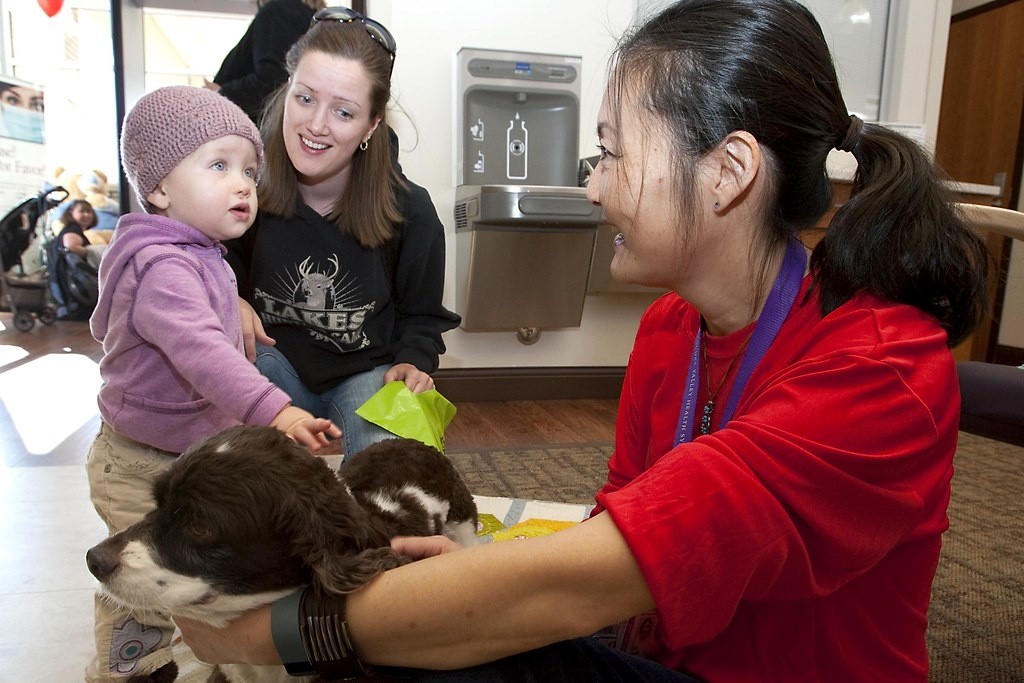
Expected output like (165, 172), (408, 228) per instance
(310, 6), (397, 85)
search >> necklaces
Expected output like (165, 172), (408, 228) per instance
(700, 325), (758, 436)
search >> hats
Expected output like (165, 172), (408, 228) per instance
(119, 86), (265, 215)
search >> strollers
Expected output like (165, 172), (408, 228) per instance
(0, 187), (70, 332)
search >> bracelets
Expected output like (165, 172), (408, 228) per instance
(266, 586), (376, 680)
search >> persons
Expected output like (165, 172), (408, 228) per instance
(44, 200), (100, 320)
(221, 6), (465, 465)
(186, 0), (328, 130)
(167, 2), (998, 682)
(0, 81), (44, 144)
(80, 86), (341, 683)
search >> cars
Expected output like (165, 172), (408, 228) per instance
(43, 203), (126, 253)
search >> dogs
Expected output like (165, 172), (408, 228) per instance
(84, 425), (481, 683)
